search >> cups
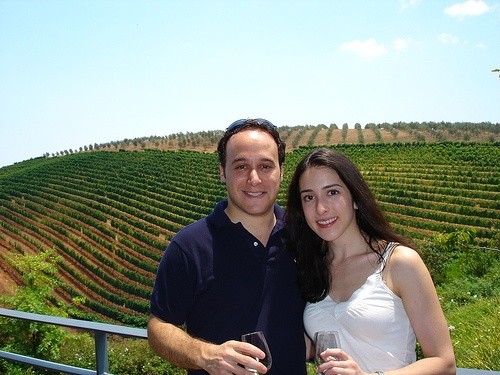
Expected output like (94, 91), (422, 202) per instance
(241, 331), (272, 375)
(313, 330), (342, 375)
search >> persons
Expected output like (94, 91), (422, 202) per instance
(287, 148), (456, 375)
(147, 118), (307, 375)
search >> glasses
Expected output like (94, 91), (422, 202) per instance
(226, 119), (277, 136)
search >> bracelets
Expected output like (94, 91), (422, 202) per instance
(374, 370), (385, 375)
